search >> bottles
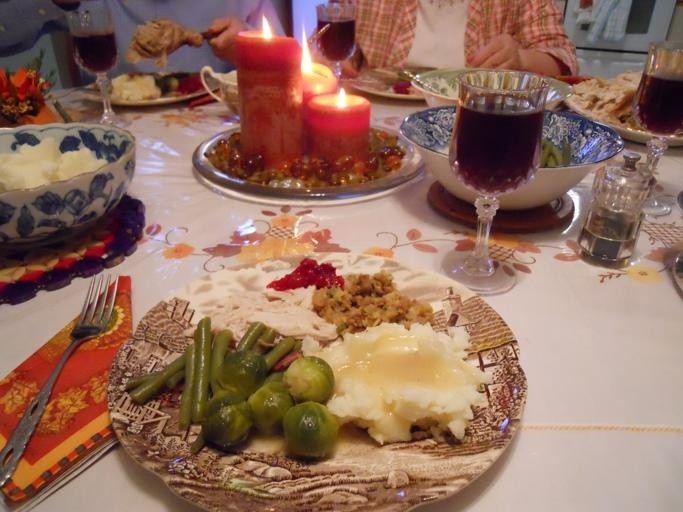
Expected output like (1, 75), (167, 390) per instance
(576, 150), (654, 269)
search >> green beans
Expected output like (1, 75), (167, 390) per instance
(543, 136), (570, 167)
(128, 318), (296, 427)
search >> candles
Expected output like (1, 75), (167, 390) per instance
(234, 32), (369, 176)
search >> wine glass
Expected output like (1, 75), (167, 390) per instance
(627, 41), (683, 219)
(439, 70), (552, 298)
(65, 7), (133, 130)
(315, 3), (357, 82)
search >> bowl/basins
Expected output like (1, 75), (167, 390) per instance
(395, 104), (626, 214)
(0, 120), (138, 258)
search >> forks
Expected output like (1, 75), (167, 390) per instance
(1, 273), (121, 490)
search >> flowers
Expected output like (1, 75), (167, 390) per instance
(0, 53), (66, 128)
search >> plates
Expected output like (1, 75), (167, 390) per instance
(77, 68), (220, 107)
(409, 67), (575, 110)
(104, 250), (528, 511)
(347, 72), (428, 102)
(564, 70), (683, 149)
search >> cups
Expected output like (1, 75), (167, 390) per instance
(199, 63), (240, 118)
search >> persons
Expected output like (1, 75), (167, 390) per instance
(0, 0), (287, 85)
(311, 0), (580, 82)
